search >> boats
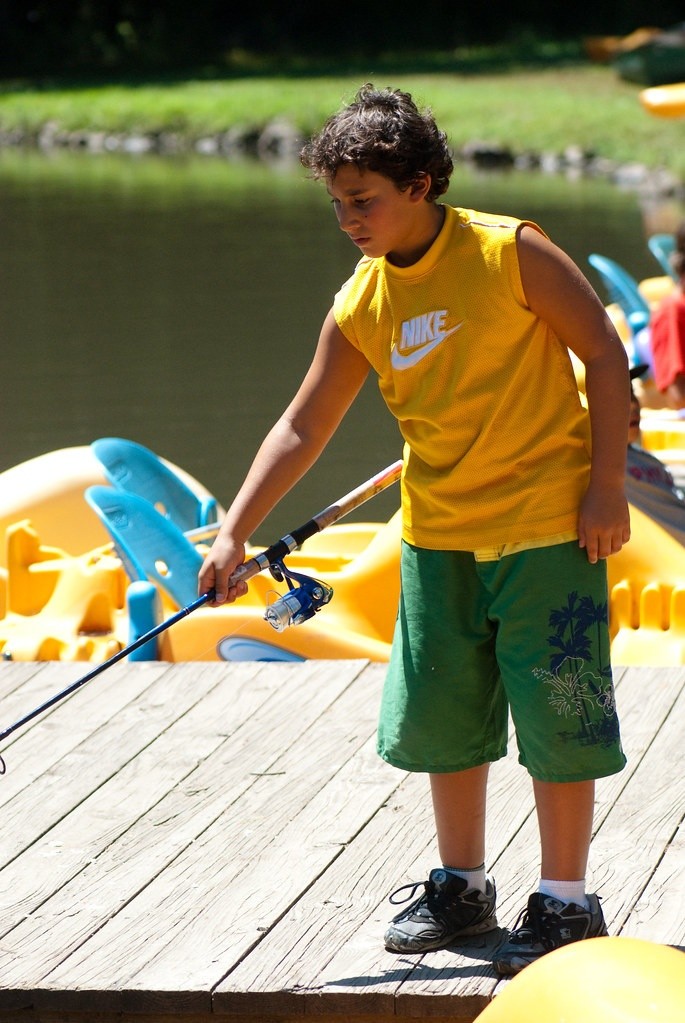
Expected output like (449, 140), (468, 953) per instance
(0, 233), (684, 665)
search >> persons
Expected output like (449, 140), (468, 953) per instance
(626, 221), (685, 544)
(197, 82), (631, 974)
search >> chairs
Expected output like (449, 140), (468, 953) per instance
(86, 487), (213, 613)
(587, 254), (652, 377)
(648, 233), (681, 283)
(89, 439), (218, 553)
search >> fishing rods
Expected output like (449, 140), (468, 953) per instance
(0, 454), (410, 778)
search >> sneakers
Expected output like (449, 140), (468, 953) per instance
(501, 891), (608, 978)
(384, 864), (500, 952)
